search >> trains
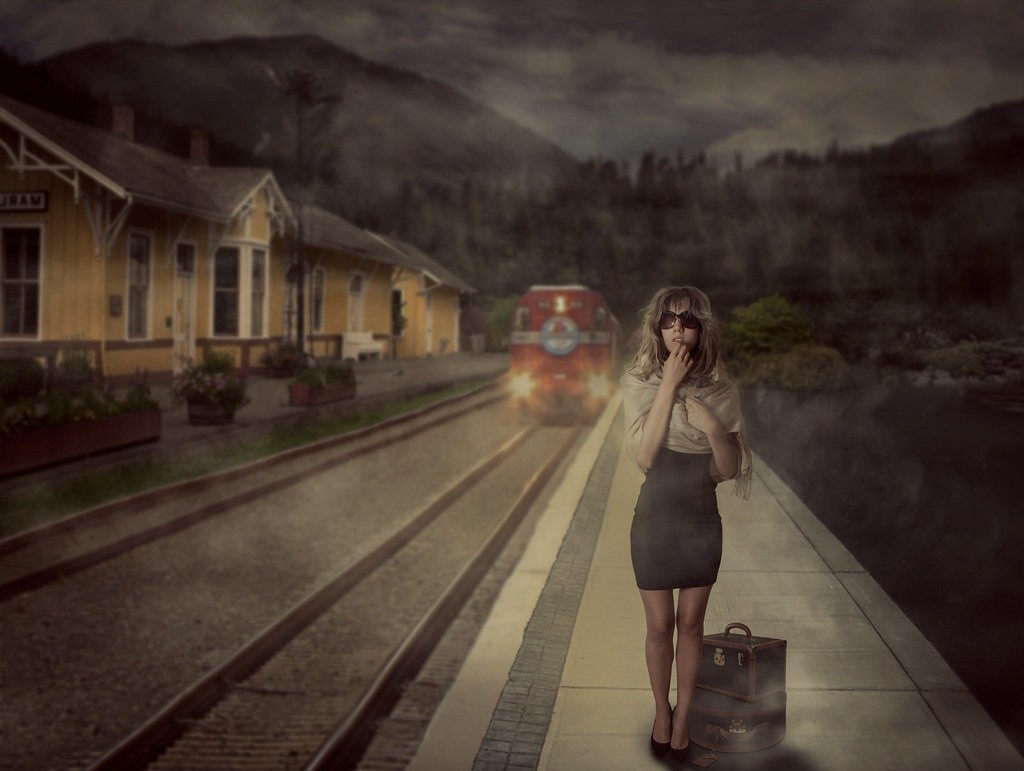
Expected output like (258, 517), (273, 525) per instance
(511, 284), (624, 428)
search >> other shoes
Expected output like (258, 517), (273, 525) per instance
(670, 705), (690, 761)
(651, 702), (673, 757)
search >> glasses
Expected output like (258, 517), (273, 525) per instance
(659, 309), (701, 330)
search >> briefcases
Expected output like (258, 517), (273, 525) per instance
(696, 622), (787, 701)
(689, 687), (787, 753)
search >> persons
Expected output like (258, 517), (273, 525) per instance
(621, 285), (754, 764)
